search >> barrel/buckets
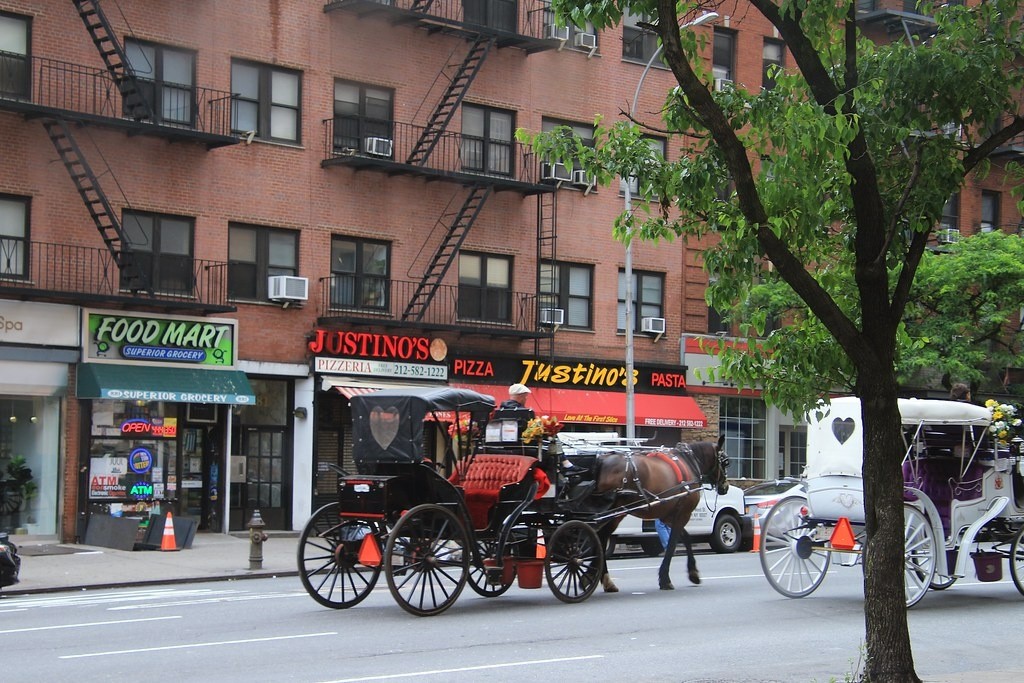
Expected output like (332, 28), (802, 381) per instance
(513, 559), (545, 589)
(946, 532), (1003, 582)
(482, 555), (514, 585)
(111, 503), (123, 517)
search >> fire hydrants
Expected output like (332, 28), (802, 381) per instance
(246, 509), (267, 569)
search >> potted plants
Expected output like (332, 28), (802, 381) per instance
(367, 257), (388, 306)
(6, 457), (33, 511)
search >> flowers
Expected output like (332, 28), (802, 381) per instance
(522, 415), (561, 443)
(448, 421), (485, 447)
(984, 397), (1023, 446)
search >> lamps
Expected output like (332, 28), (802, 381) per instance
(29, 399), (38, 423)
(294, 407), (309, 419)
(9, 396), (18, 423)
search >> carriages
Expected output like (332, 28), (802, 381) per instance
(298, 384), (727, 616)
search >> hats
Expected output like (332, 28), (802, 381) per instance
(509, 383), (532, 395)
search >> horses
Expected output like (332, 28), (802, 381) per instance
(579, 434), (729, 592)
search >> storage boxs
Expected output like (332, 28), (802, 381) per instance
(337, 474), (408, 512)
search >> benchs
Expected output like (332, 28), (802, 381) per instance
(453, 453), (549, 502)
(353, 452), (461, 518)
(902, 450), (1012, 523)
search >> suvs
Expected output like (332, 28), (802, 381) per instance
(540, 446), (745, 560)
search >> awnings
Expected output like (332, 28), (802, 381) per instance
(335, 383), (709, 429)
(76, 362), (256, 405)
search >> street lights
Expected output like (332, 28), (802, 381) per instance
(622, 13), (723, 448)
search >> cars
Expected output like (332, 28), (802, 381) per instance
(743, 479), (820, 548)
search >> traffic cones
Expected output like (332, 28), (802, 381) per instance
(156, 511), (180, 551)
(749, 514), (768, 552)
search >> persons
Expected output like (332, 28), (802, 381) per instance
(500, 383), (589, 475)
(945, 383), (976, 451)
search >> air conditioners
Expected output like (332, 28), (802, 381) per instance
(366, 136), (392, 156)
(541, 308), (564, 324)
(542, 162), (573, 182)
(575, 33), (597, 50)
(574, 169), (597, 187)
(545, 24), (570, 41)
(940, 228), (962, 243)
(269, 275), (310, 302)
(642, 318), (667, 334)
(715, 79), (734, 93)
